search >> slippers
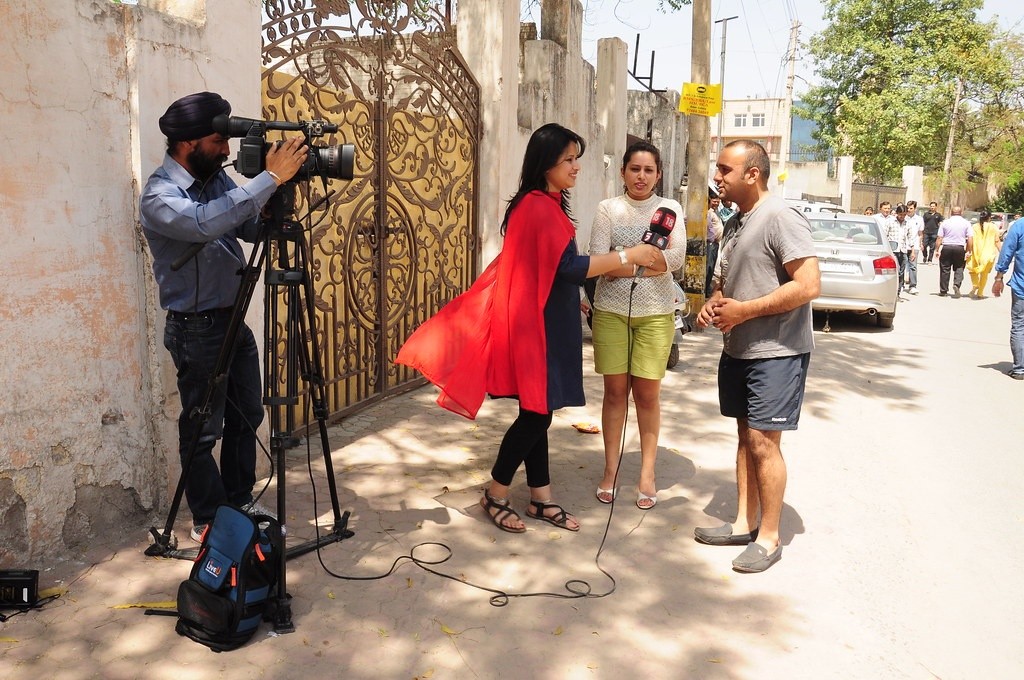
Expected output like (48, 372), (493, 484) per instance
(597, 485), (617, 503)
(636, 488), (657, 510)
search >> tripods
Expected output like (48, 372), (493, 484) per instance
(145, 182), (356, 635)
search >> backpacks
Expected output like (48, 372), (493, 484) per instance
(176, 503), (283, 651)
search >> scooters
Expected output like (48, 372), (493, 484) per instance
(583, 240), (692, 370)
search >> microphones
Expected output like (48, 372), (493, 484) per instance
(631, 207), (676, 291)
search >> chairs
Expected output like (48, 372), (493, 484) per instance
(813, 230), (834, 240)
(853, 233), (877, 244)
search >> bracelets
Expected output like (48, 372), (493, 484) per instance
(632, 264), (636, 275)
(267, 170), (281, 182)
(995, 277), (1003, 281)
(619, 250), (628, 265)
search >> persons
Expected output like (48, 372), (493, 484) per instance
(935, 207), (974, 298)
(589, 142), (687, 509)
(966, 209), (1003, 298)
(923, 202), (943, 266)
(706, 193), (736, 298)
(992, 217), (1024, 380)
(693, 139), (821, 572)
(865, 200), (924, 301)
(394, 123), (660, 533)
(138, 92), (309, 543)
(1007, 212), (1020, 233)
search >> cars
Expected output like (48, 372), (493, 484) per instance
(792, 198), (900, 327)
(972, 212), (1015, 242)
(891, 206), (945, 222)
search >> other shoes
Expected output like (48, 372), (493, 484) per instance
(1008, 370), (1024, 380)
(733, 538), (782, 573)
(898, 286), (984, 301)
(694, 523), (759, 545)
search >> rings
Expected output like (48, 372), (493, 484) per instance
(650, 263), (653, 266)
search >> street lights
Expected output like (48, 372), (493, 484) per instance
(778, 72), (820, 198)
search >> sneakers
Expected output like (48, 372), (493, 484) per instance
(190, 525), (205, 543)
(237, 501), (278, 530)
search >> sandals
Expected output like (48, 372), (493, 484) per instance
(480, 488), (526, 533)
(526, 500), (580, 531)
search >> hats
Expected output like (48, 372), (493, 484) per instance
(158, 92), (231, 140)
